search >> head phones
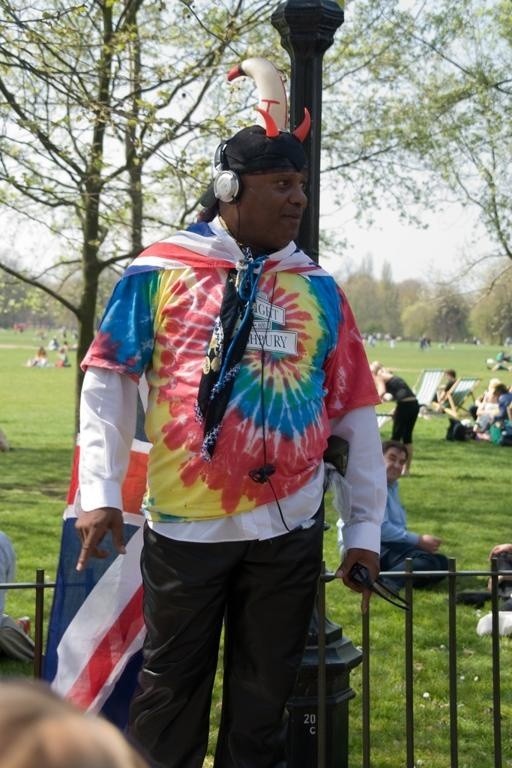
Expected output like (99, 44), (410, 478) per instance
(213, 139), (242, 204)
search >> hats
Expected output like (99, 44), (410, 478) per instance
(220, 56), (312, 175)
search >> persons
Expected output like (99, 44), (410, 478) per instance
(475, 543), (512, 611)
(336, 441), (452, 599)
(357, 329), (512, 479)
(15, 323), (78, 370)
(0, 530), (154, 768)
(72, 124), (390, 768)
